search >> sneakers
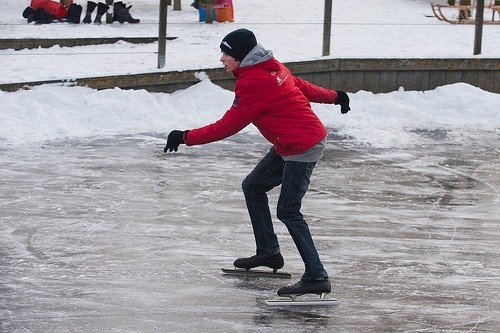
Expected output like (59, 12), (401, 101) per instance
(233, 252), (284, 273)
(277, 278), (331, 297)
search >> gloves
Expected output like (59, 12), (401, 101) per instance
(334, 90), (351, 114)
(164, 130), (184, 153)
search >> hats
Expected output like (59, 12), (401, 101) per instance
(220, 28), (257, 63)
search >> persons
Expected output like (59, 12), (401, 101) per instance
(163, 29), (351, 297)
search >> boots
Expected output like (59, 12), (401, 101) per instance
(113, 1), (139, 24)
(83, 1), (97, 24)
(93, 2), (110, 25)
